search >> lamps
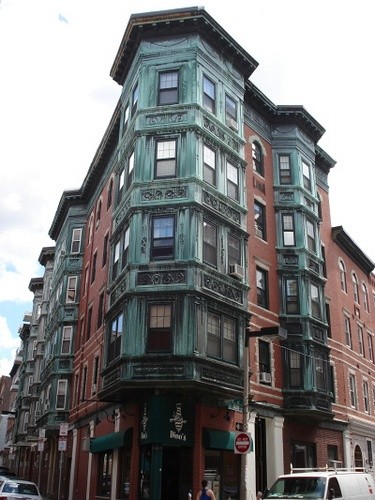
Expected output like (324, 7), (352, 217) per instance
(248, 409), (262, 422)
(74, 399), (118, 430)
(364, 458), (373, 467)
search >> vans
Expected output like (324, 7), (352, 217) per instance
(256, 463), (375, 500)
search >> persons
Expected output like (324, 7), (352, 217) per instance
(256, 491), (263, 500)
(196, 479), (216, 500)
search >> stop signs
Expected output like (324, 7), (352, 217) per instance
(235, 433), (250, 453)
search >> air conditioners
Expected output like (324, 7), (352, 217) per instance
(260, 373), (271, 383)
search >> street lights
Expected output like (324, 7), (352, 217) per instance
(239, 325), (288, 500)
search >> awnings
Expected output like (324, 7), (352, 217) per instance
(90, 426), (133, 454)
(203, 428), (237, 450)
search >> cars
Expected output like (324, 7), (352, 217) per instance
(0, 465), (44, 500)
